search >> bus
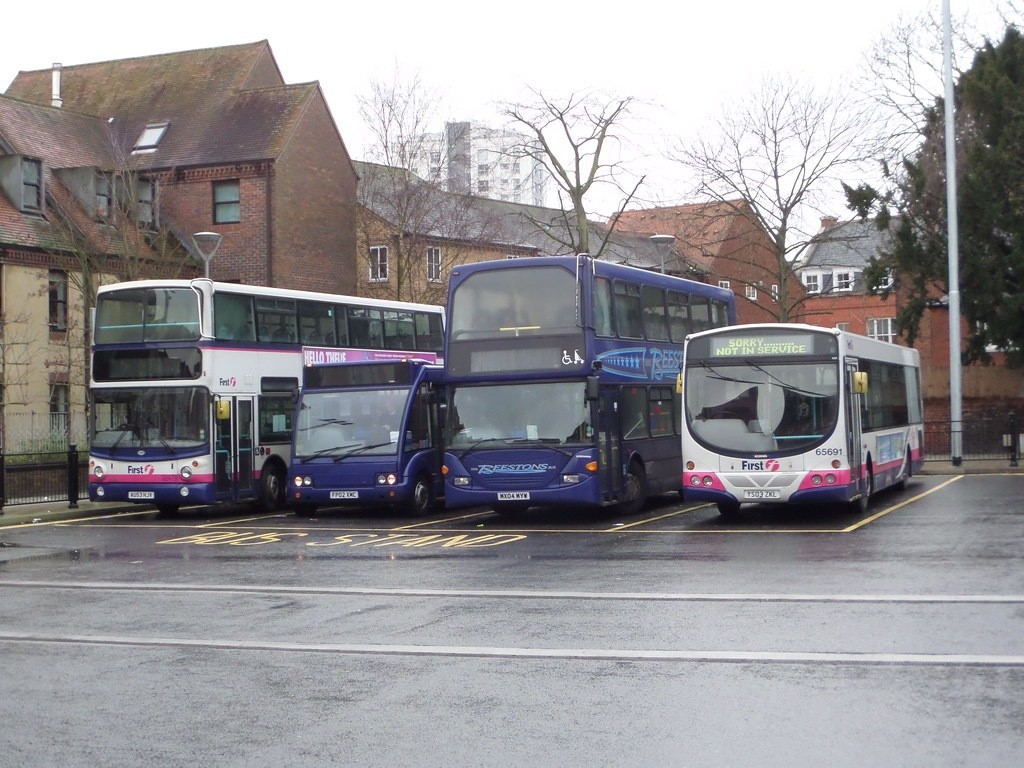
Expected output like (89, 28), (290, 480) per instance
(443, 253), (735, 517)
(676, 323), (925, 517)
(88, 277), (446, 515)
(284, 358), (445, 517)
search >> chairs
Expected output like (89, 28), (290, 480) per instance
(215, 321), (431, 351)
(621, 309), (722, 340)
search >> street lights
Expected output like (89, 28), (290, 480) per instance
(192, 230), (222, 278)
(650, 234), (676, 273)
